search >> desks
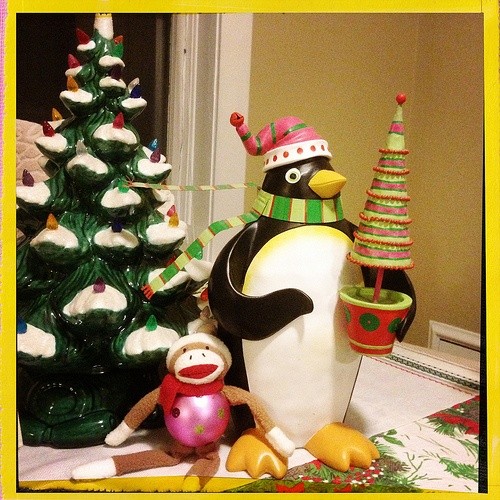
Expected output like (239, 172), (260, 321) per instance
(19, 341), (479, 493)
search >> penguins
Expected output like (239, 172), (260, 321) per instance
(208, 112), (416, 481)
